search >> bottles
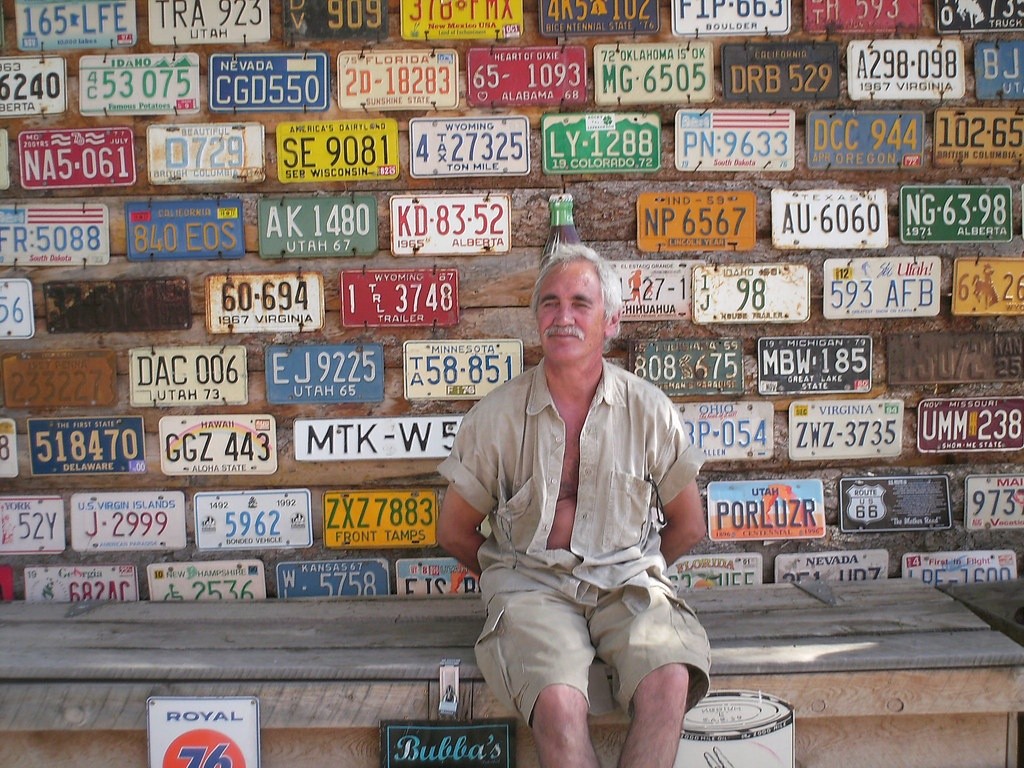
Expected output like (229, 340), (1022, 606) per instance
(540, 192), (584, 258)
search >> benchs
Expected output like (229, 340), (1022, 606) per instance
(0, 592), (1024, 768)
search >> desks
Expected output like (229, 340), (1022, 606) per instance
(940, 579), (1024, 643)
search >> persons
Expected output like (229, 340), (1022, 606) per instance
(434, 245), (710, 768)
